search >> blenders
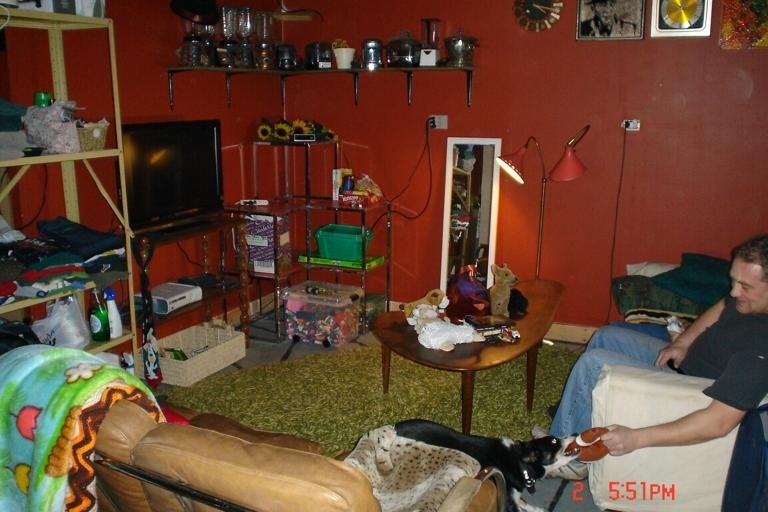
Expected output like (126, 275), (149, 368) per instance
(220, 6), (276, 69)
(418, 17), (442, 66)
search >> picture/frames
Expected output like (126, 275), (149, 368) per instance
(575, 0), (646, 42)
(650, 0), (712, 37)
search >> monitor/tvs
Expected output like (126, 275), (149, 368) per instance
(114, 120), (225, 235)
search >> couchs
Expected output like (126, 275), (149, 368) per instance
(1, 329), (507, 510)
(592, 260), (767, 512)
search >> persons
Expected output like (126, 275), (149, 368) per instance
(528, 232), (768, 481)
(580, 1), (639, 37)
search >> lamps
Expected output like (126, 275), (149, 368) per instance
(495, 123), (594, 347)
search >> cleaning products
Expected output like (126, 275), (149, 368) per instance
(88, 289), (110, 340)
(103, 288), (122, 338)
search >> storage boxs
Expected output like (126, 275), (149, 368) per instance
(234, 213), (386, 347)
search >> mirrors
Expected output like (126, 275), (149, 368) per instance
(440, 137), (502, 295)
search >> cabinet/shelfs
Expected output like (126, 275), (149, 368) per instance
(130, 206), (252, 351)
(0, 0), (150, 398)
(221, 132), (393, 340)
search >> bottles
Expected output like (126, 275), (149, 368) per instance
(187, 21), (202, 67)
(343, 167), (353, 190)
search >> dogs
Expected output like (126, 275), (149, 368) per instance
(351, 418), (582, 512)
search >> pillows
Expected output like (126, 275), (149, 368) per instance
(612, 254), (735, 315)
(343, 424), (484, 511)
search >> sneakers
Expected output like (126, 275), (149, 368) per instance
(531, 426), (588, 482)
